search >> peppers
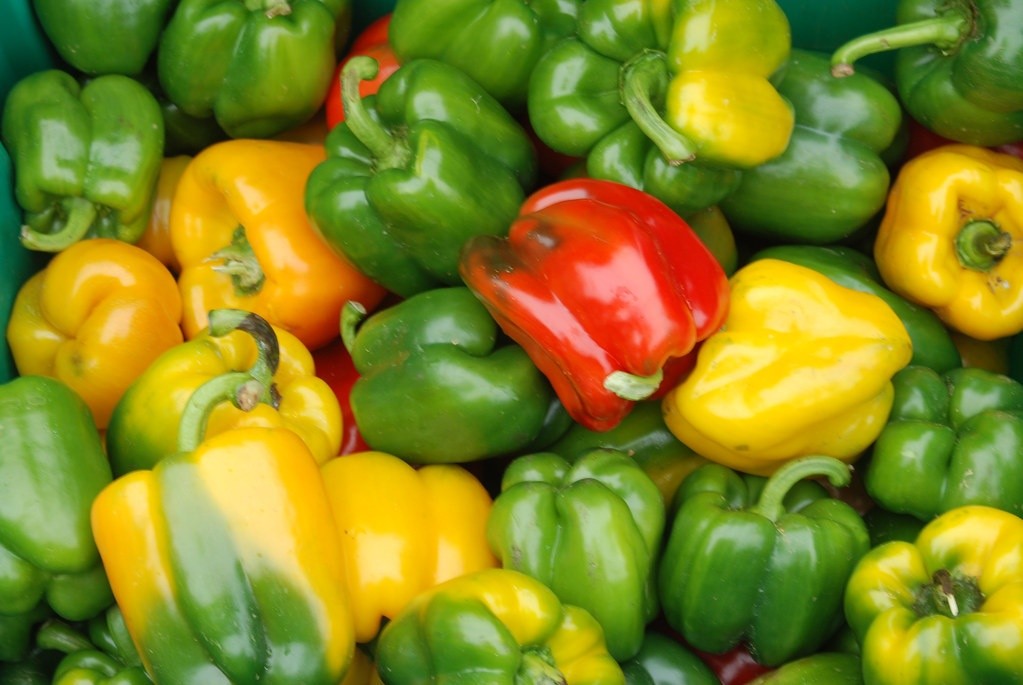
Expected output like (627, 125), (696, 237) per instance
(0, 0), (1023, 685)
